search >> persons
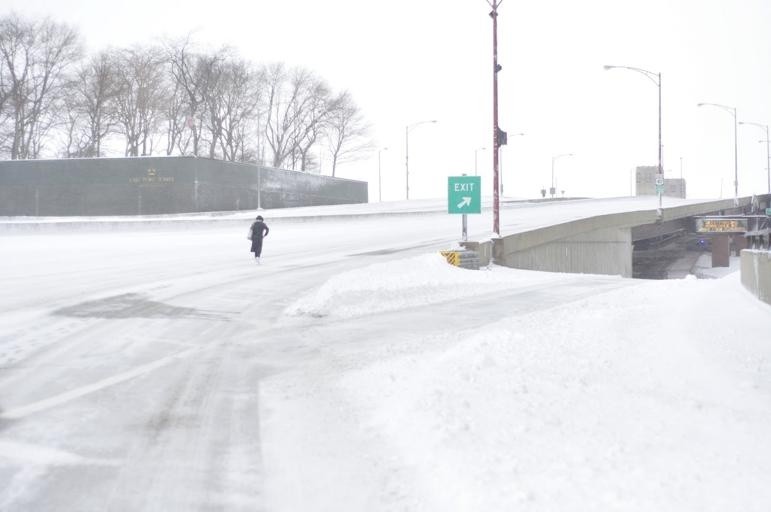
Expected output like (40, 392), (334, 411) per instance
(248, 215), (269, 263)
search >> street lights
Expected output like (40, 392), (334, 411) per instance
(735, 119), (770, 199)
(499, 132), (527, 195)
(550, 152), (575, 198)
(255, 99), (298, 212)
(475, 147), (488, 176)
(695, 102), (739, 206)
(405, 119), (439, 201)
(601, 64), (665, 225)
(377, 147), (390, 203)
